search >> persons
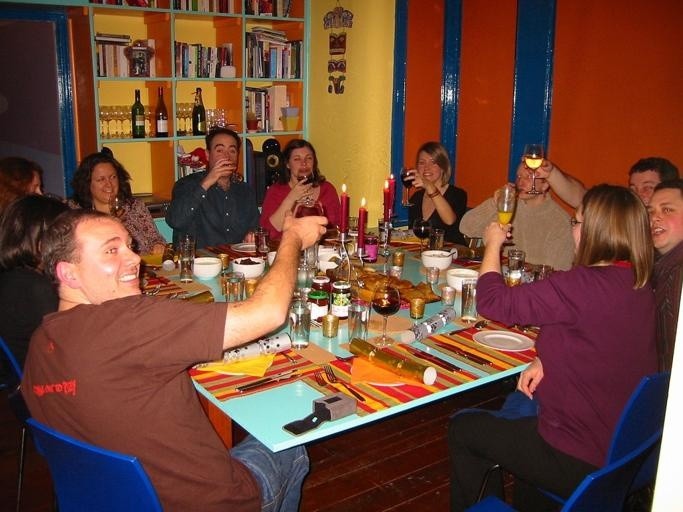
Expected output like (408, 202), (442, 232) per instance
(522, 153), (678, 208)
(646, 179), (682, 372)
(404, 142), (466, 245)
(447, 183), (659, 511)
(0, 158), (42, 216)
(165, 126), (261, 249)
(67, 151), (170, 255)
(2, 197), (71, 375)
(260, 137), (342, 238)
(21, 209), (330, 512)
(460, 156), (577, 273)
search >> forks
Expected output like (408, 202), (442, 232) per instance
(325, 365), (367, 402)
(313, 371), (359, 403)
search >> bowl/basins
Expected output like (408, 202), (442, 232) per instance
(266, 252), (278, 265)
(232, 257), (266, 276)
(421, 250), (453, 271)
(446, 269), (480, 290)
(318, 254), (340, 272)
(191, 257), (223, 280)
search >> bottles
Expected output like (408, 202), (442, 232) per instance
(361, 235), (378, 263)
(86, 86), (234, 140)
(311, 276), (331, 294)
(305, 290), (328, 321)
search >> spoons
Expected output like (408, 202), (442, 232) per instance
(448, 320), (488, 337)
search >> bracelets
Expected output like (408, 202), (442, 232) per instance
(426, 188), (441, 200)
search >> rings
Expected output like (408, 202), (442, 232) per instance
(303, 189), (308, 194)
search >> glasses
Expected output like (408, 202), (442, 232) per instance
(570, 217), (583, 227)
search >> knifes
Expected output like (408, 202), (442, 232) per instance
(236, 368), (298, 392)
(239, 373), (304, 393)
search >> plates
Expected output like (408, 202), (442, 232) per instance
(474, 330), (534, 352)
(232, 243), (271, 253)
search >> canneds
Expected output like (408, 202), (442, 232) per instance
(311, 276), (330, 311)
(306, 290), (330, 328)
(364, 235), (378, 263)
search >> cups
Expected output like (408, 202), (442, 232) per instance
(393, 251), (404, 266)
(441, 287), (456, 306)
(254, 226), (269, 253)
(377, 217), (386, 232)
(181, 256), (193, 283)
(402, 305), (456, 343)
(391, 266), (402, 278)
(323, 315), (339, 337)
(162, 243), (176, 272)
(537, 263), (552, 273)
(181, 234), (195, 256)
(509, 250), (525, 270)
(348, 337), (377, 360)
(348, 216), (358, 230)
(435, 229), (444, 248)
(246, 280), (256, 297)
(348, 305), (369, 343)
(425, 266), (439, 285)
(410, 299), (425, 320)
(224, 272), (244, 302)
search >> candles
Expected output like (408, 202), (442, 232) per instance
(383, 177), (395, 222)
(358, 208), (367, 247)
(340, 194), (348, 231)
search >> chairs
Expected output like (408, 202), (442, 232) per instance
(463, 370), (669, 512)
(0, 341), (160, 512)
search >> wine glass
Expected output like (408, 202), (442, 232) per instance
(297, 168), (317, 208)
(108, 195), (126, 218)
(413, 220), (431, 250)
(496, 186), (517, 245)
(225, 156), (243, 184)
(295, 204), (322, 271)
(523, 144), (545, 196)
(400, 167), (416, 207)
(370, 286), (401, 346)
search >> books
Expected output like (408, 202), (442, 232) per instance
(90, 1), (303, 131)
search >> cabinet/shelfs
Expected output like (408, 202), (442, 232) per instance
(67, 0), (311, 213)
